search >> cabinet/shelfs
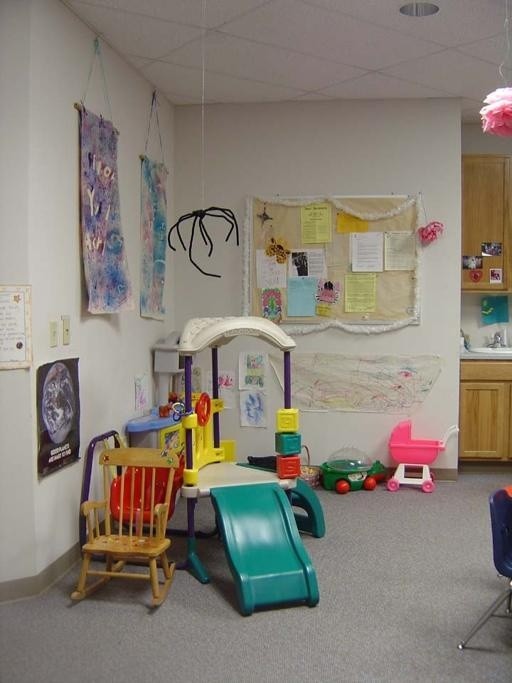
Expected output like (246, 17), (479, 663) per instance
(462, 155), (512, 292)
(459, 358), (511, 461)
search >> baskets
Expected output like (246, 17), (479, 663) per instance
(300, 445), (323, 489)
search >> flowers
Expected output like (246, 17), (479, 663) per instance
(478, 85), (512, 138)
(418, 221), (443, 243)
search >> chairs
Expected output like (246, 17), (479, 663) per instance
(455, 488), (511, 650)
(69, 445), (181, 609)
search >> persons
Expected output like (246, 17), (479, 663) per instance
(100, 162), (115, 178)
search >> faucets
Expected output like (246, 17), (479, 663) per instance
(493, 332), (503, 347)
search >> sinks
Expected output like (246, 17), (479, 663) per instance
(470, 347), (512, 354)
